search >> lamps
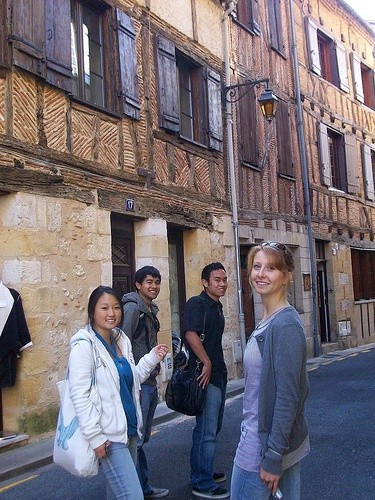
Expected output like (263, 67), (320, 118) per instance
(225, 78), (278, 124)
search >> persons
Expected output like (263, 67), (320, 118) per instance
(230, 242), (311, 500)
(118, 265), (169, 500)
(180, 261), (229, 499)
(67, 285), (168, 500)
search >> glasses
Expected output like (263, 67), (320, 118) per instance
(261, 241), (286, 252)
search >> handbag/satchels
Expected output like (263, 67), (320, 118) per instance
(165, 354), (208, 416)
(52, 344), (105, 478)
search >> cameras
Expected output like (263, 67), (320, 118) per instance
(272, 486), (283, 499)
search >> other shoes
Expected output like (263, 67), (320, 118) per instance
(213, 473), (227, 483)
(143, 487), (169, 498)
(192, 486), (230, 499)
